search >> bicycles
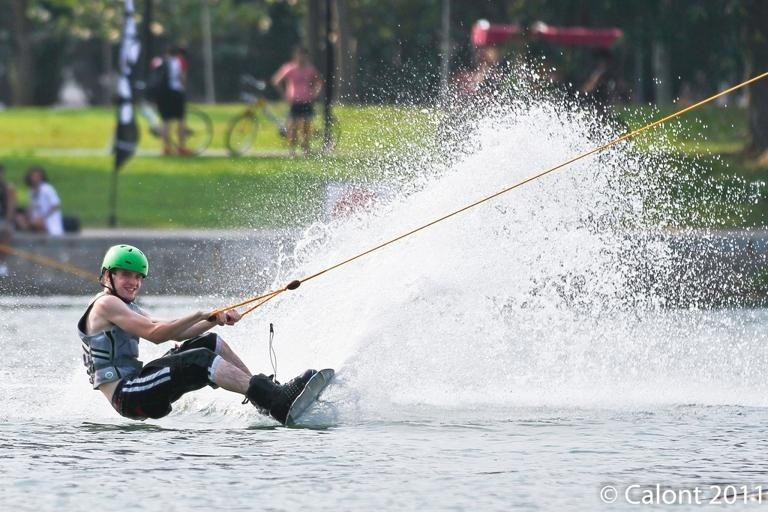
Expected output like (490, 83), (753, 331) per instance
(224, 75), (340, 159)
(134, 96), (214, 154)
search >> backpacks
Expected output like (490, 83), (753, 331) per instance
(144, 64), (169, 102)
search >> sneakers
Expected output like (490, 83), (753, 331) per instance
(273, 367), (317, 424)
(163, 145), (194, 155)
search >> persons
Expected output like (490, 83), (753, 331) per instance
(0, 165), (83, 279)
(158, 36), (199, 156)
(271, 44), (325, 158)
(75, 244), (337, 428)
(447, 30), (633, 144)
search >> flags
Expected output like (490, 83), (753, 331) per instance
(115, 1), (144, 169)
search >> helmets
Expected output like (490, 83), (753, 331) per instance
(100, 243), (148, 278)
